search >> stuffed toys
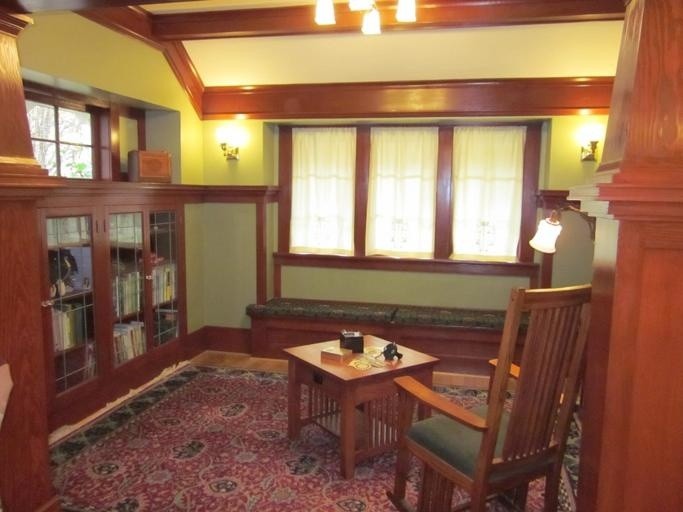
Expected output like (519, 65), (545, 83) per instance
(47, 249), (77, 300)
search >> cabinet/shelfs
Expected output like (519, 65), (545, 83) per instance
(35, 182), (181, 431)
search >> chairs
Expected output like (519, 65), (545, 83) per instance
(388, 286), (607, 512)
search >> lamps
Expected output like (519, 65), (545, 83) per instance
(528, 209), (562, 254)
(315, 0), (416, 34)
(218, 124), (249, 161)
(574, 123), (606, 162)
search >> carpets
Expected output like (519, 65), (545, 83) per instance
(50, 364), (581, 512)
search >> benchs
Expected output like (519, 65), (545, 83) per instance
(245, 252), (540, 353)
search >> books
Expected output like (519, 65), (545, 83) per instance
(51, 263), (180, 395)
